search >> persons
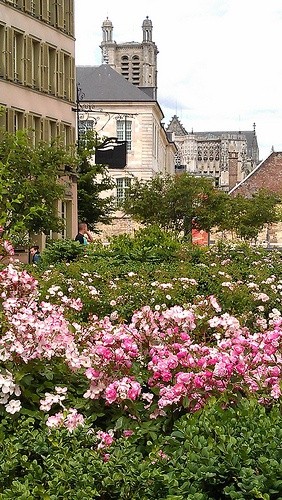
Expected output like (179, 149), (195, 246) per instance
(30, 246), (41, 264)
(74, 223), (93, 245)
